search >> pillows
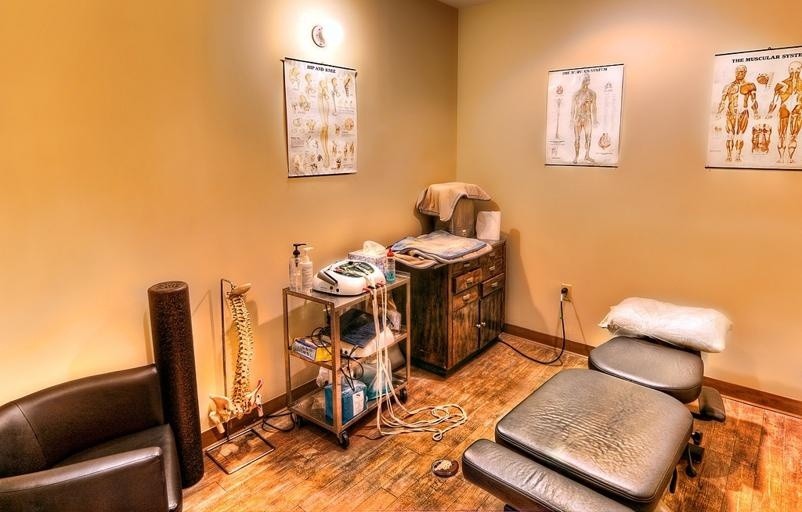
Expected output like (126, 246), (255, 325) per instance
(599, 297), (732, 354)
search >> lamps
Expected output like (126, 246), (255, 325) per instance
(312, 24), (346, 48)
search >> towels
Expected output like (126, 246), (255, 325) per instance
(417, 181), (492, 222)
(385, 229), (494, 270)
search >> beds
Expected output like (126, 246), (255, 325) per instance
(462, 337), (726, 511)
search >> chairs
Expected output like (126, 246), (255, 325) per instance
(0, 365), (184, 511)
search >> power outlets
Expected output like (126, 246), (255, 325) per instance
(560, 283), (572, 302)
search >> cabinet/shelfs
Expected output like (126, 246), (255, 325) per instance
(385, 241), (509, 376)
(282, 269), (410, 442)
